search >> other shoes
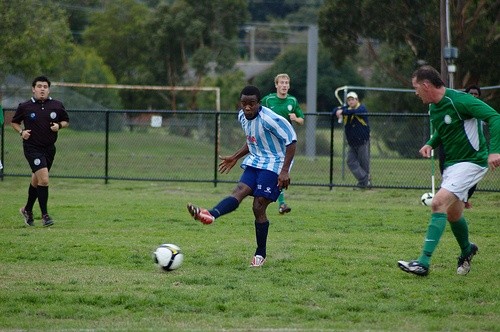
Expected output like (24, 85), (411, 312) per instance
(359, 175), (371, 188)
(464, 202), (472, 208)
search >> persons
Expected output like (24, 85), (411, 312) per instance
(333, 92), (372, 190)
(260, 73), (305, 215)
(10, 76), (70, 227)
(187, 86), (297, 267)
(437, 85), (481, 210)
(396, 65), (500, 276)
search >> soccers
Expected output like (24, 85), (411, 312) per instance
(421, 193), (434, 207)
(154, 244), (183, 271)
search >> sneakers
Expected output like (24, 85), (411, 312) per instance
(456, 244), (479, 275)
(279, 203), (291, 214)
(21, 207), (33, 226)
(396, 260), (429, 277)
(249, 254), (267, 267)
(42, 214), (54, 227)
(187, 202), (216, 225)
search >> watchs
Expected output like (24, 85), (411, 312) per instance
(19, 129), (24, 136)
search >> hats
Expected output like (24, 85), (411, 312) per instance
(346, 92), (358, 98)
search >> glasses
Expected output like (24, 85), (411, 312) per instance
(470, 92), (479, 95)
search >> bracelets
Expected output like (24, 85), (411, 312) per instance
(58, 122), (63, 130)
